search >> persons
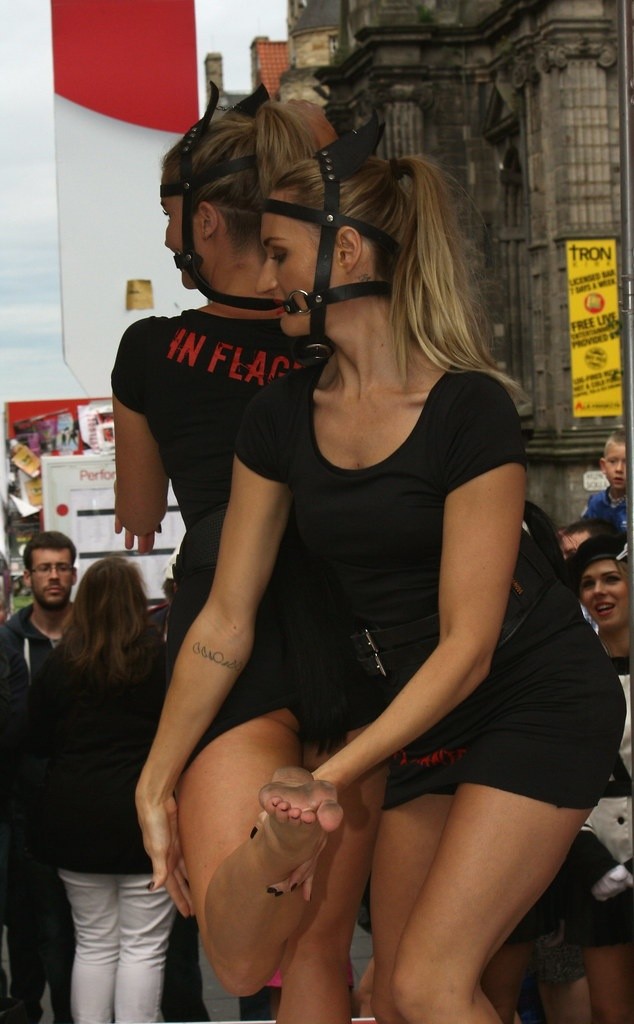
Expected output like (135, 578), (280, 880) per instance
(134, 130), (623, 1024)
(113, 79), (389, 1024)
(0, 430), (632, 1024)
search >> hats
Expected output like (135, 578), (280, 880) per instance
(575, 535), (629, 584)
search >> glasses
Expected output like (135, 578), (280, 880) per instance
(30, 562), (74, 578)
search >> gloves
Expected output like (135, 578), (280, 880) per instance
(589, 866), (634, 902)
